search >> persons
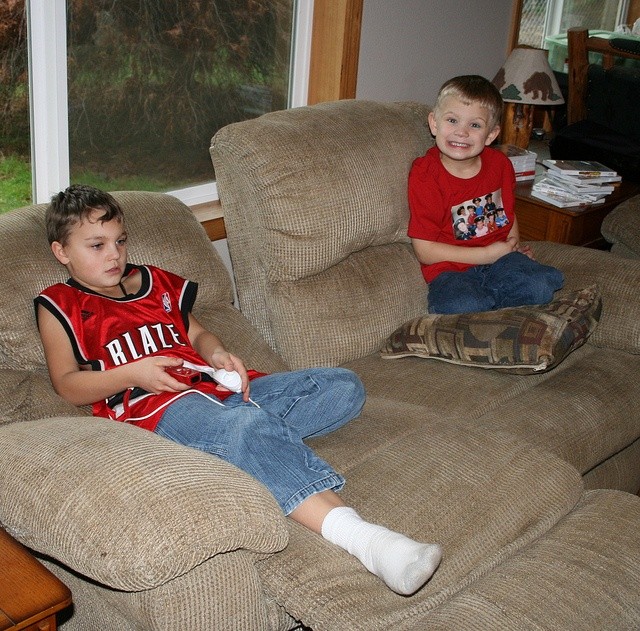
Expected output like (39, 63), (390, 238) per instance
(410, 75), (563, 314)
(34, 183), (443, 597)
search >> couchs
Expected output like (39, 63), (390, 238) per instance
(600, 194), (640, 260)
(0, 99), (640, 630)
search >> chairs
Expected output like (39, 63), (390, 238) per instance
(568, 27), (640, 127)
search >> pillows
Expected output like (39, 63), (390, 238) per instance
(379, 284), (604, 376)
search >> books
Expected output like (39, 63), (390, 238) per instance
(493, 141), (537, 182)
(531, 159), (622, 208)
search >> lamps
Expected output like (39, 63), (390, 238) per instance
(490, 45), (565, 146)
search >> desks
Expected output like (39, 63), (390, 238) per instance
(516, 182), (626, 251)
(0, 525), (72, 630)
